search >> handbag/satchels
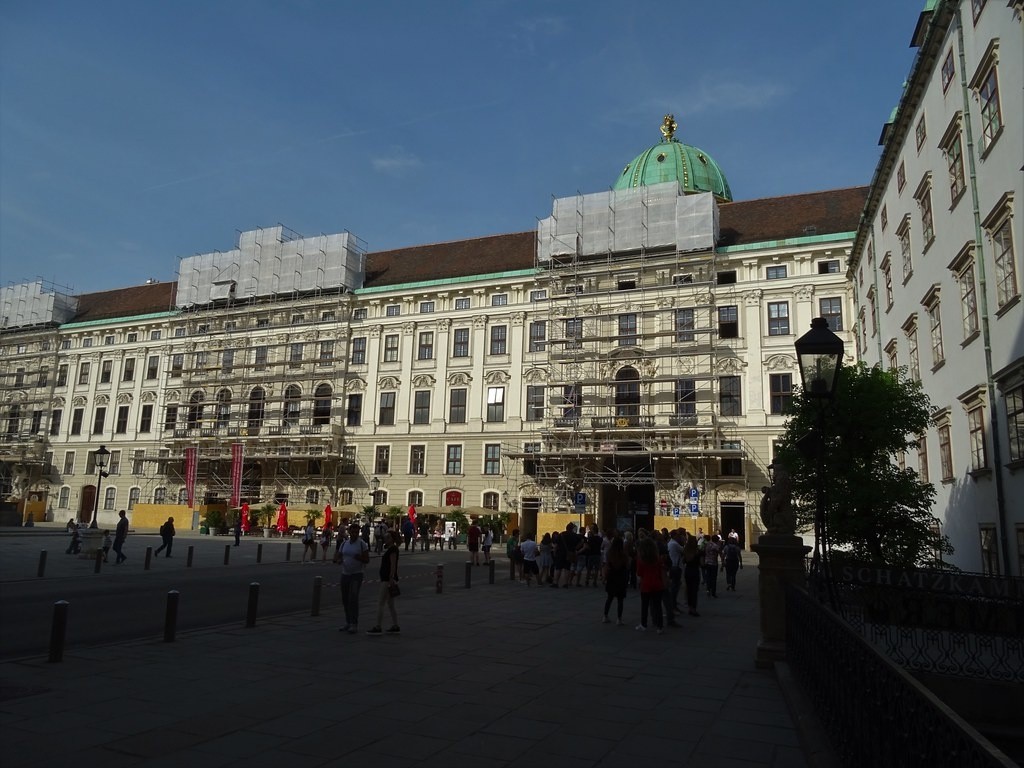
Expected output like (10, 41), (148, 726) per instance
(566, 551), (578, 563)
(668, 565), (682, 579)
(390, 582), (400, 598)
(302, 534), (308, 544)
(320, 537), (328, 546)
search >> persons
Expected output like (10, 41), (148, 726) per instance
(467, 519), (493, 565)
(67, 518), (76, 532)
(302, 520), (314, 564)
(113, 510), (129, 566)
(321, 522), (332, 559)
(102, 529), (112, 563)
(336, 516), (442, 562)
(154, 517), (175, 558)
(507, 522), (743, 635)
(333, 524), (370, 634)
(448, 523), (457, 550)
(366, 531), (402, 635)
(233, 520), (241, 546)
(66, 525), (79, 553)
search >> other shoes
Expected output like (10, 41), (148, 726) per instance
(667, 620), (683, 628)
(707, 591), (710, 598)
(519, 577), (598, 588)
(616, 618), (625, 625)
(385, 625), (401, 633)
(672, 608), (680, 615)
(688, 610), (701, 617)
(602, 617), (611, 623)
(635, 624), (648, 632)
(656, 626), (664, 634)
(711, 594), (718, 598)
(339, 622), (350, 632)
(366, 627), (382, 635)
(726, 584), (735, 592)
(347, 624), (358, 633)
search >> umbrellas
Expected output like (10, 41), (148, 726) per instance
(241, 503), (249, 535)
(409, 504), (416, 523)
(324, 506), (332, 530)
(277, 505), (288, 537)
